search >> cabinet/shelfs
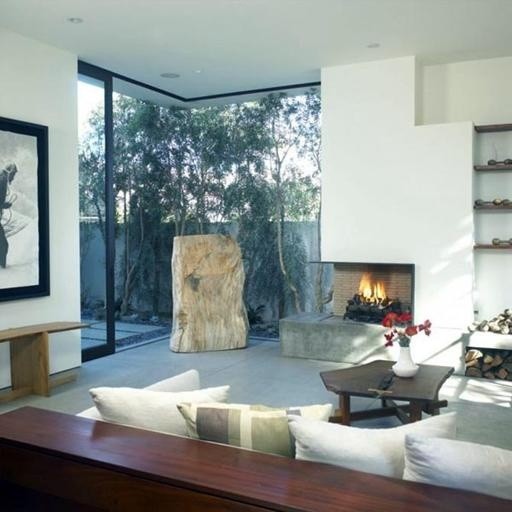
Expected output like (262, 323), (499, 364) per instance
(473, 123), (512, 249)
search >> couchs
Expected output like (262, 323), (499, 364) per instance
(75, 369), (512, 500)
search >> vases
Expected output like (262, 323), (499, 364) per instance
(391, 347), (419, 378)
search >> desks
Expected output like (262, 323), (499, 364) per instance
(0, 321), (90, 404)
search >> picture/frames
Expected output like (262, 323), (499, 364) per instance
(0, 116), (50, 302)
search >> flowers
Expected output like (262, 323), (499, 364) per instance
(382, 312), (431, 347)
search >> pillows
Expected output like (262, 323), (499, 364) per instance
(87, 385), (231, 438)
(402, 433), (512, 500)
(287, 412), (458, 480)
(176, 403), (333, 458)
